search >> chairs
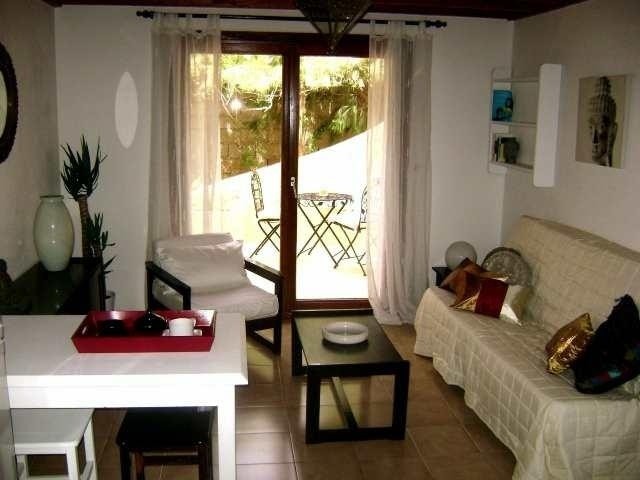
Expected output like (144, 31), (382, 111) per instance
(334, 183), (368, 276)
(249, 171), (282, 257)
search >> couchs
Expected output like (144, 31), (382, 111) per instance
(413, 216), (640, 480)
(146, 233), (284, 356)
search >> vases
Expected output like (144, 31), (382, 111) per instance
(33, 196), (75, 271)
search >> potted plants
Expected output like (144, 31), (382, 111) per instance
(60, 134), (117, 313)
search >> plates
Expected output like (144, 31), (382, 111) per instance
(322, 321), (369, 344)
(161, 328), (203, 336)
(69, 309), (217, 353)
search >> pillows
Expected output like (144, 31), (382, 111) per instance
(438, 257), (486, 292)
(464, 278), (527, 328)
(450, 272), (484, 307)
(545, 313), (593, 375)
(573, 293), (640, 393)
(155, 240), (247, 295)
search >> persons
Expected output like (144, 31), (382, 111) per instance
(589, 77), (618, 167)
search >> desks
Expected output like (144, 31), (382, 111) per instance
(297, 193), (351, 265)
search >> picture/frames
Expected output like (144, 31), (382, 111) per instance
(574, 74), (629, 168)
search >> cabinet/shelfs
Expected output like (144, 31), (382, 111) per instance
(487, 62), (562, 187)
(3, 255), (105, 315)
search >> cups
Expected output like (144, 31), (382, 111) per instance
(168, 317), (193, 336)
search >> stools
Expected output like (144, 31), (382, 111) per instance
(1, 410), (95, 480)
(116, 408), (213, 480)
